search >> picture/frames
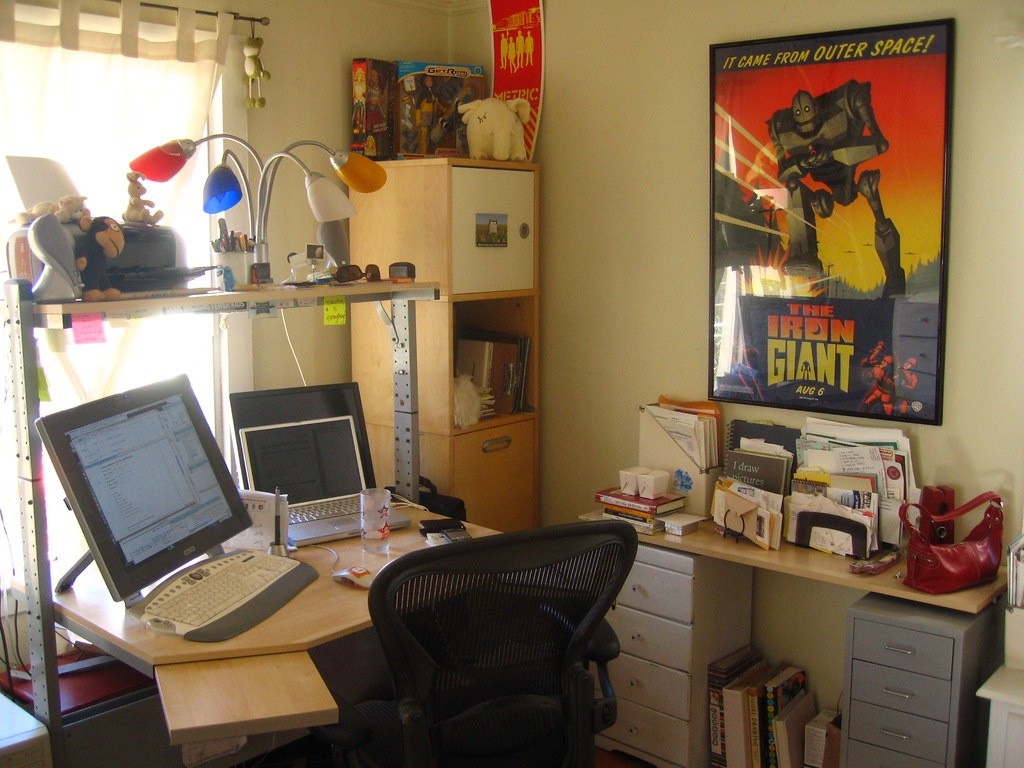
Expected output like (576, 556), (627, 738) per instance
(700, 16), (962, 425)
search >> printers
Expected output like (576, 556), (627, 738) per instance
(6, 225), (219, 294)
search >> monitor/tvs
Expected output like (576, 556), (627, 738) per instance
(230, 382), (377, 494)
(35, 373), (253, 608)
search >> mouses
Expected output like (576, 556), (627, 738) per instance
(331, 566), (374, 590)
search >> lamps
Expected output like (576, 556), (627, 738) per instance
(130, 135), (390, 288)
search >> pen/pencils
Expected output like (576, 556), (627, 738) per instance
(210, 231), (256, 252)
(275, 485), (280, 545)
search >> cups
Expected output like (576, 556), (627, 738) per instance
(214, 252), (255, 292)
(360, 487), (391, 554)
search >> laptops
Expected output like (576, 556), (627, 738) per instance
(239, 416), (411, 547)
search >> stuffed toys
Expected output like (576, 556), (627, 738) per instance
(122, 171), (163, 223)
(458, 98), (531, 160)
(17, 196), (90, 224)
(74, 215), (126, 301)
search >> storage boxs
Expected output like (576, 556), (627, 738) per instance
(349, 54), (498, 160)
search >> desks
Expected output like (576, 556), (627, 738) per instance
(578, 509), (1006, 614)
(975, 666), (1024, 768)
(45, 489), (508, 768)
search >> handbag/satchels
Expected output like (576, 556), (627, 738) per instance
(899, 492), (1004, 595)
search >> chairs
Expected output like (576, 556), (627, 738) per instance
(368, 521), (638, 768)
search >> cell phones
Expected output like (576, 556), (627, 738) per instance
(442, 528), (472, 543)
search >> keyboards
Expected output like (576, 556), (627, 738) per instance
(126, 549), (319, 642)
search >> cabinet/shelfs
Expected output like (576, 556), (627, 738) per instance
(342, 155), (549, 529)
(837, 596), (995, 768)
(584, 548), (762, 768)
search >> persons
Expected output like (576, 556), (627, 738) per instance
(415, 75), (439, 153)
(353, 68), (366, 134)
(366, 69), (386, 133)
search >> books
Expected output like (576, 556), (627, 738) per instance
(707, 644), (816, 768)
(724, 449), (788, 494)
(595, 486), (685, 535)
(456, 340), (494, 388)
(1005, 534), (1024, 608)
